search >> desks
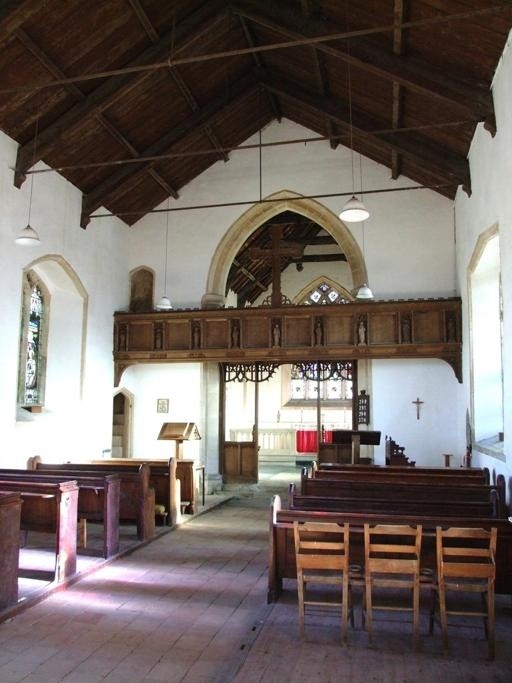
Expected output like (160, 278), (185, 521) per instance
(0, 457), (194, 613)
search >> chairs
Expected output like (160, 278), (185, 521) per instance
(363, 521), (421, 648)
(292, 519), (350, 644)
(429, 524), (499, 661)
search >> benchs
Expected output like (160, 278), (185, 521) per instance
(266, 459), (511, 604)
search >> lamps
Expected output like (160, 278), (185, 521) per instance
(153, 191), (174, 310)
(355, 147), (374, 299)
(14, 90), (39, 244)
(338, 61), (370, 222)
